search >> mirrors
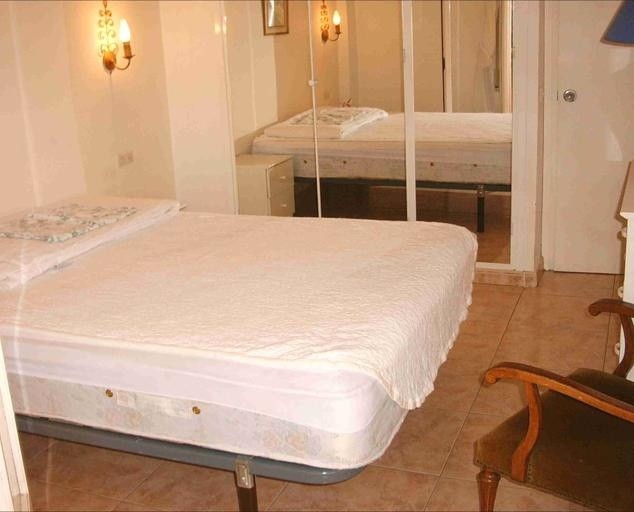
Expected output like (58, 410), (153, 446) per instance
(221, 1), (515, 265)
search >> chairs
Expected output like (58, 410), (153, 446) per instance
(473, 298), (634, 512)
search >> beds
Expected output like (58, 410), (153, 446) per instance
(0, 209), (477, 512)
(252, 111), (513, 233)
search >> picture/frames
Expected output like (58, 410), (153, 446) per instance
(261, 1), (289, 36)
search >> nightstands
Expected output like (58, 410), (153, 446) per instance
(234, 154), (295, 217)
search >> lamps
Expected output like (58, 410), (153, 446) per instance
(320, 1), (342, 44)
(96, 0), (135, 75)
